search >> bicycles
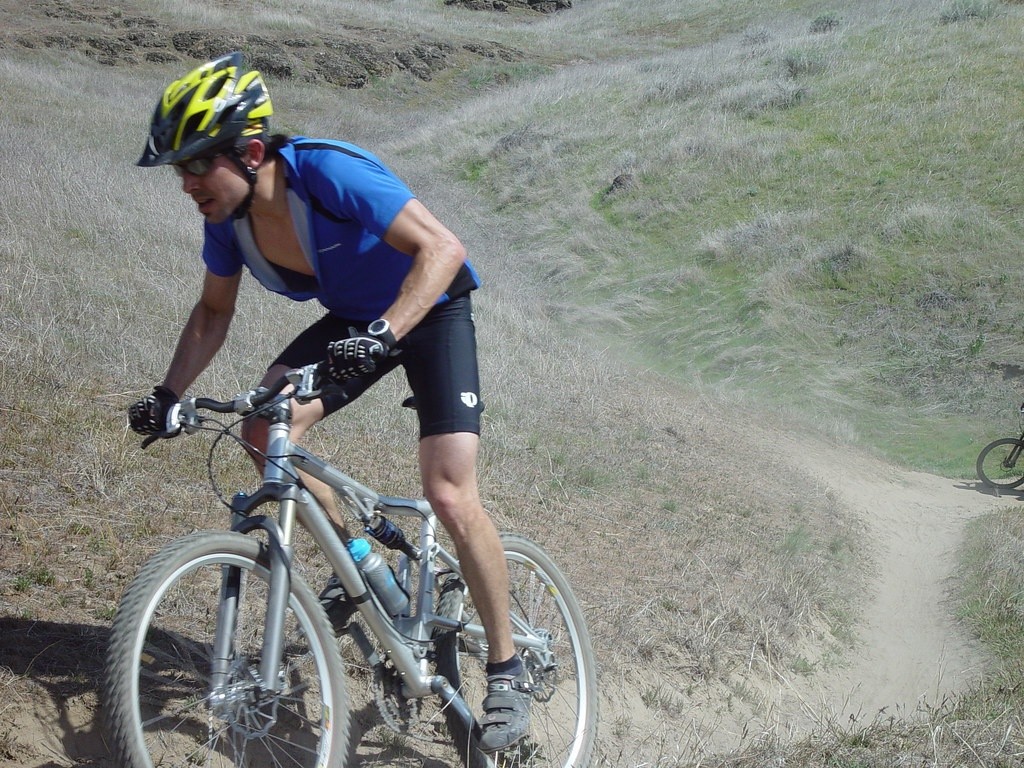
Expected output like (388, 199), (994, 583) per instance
(975, 406), (1024, 490)
(100, 360), (594, 768)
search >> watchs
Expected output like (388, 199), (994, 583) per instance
(368, 319), (397, 351)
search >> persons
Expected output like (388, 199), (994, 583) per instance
(134, 51), (534, 754)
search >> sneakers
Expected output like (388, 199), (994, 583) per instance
(477, 669), (535, 755)
(297, 572), (359, 638)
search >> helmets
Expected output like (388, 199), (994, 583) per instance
(137, 51), (272, 167)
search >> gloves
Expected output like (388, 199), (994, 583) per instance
(128, 386), (182, 439)
(326, 325), (387, 385)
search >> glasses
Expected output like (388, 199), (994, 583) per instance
(173, 145), (243, 177)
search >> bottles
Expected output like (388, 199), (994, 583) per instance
(345, 538), (410, 616)
